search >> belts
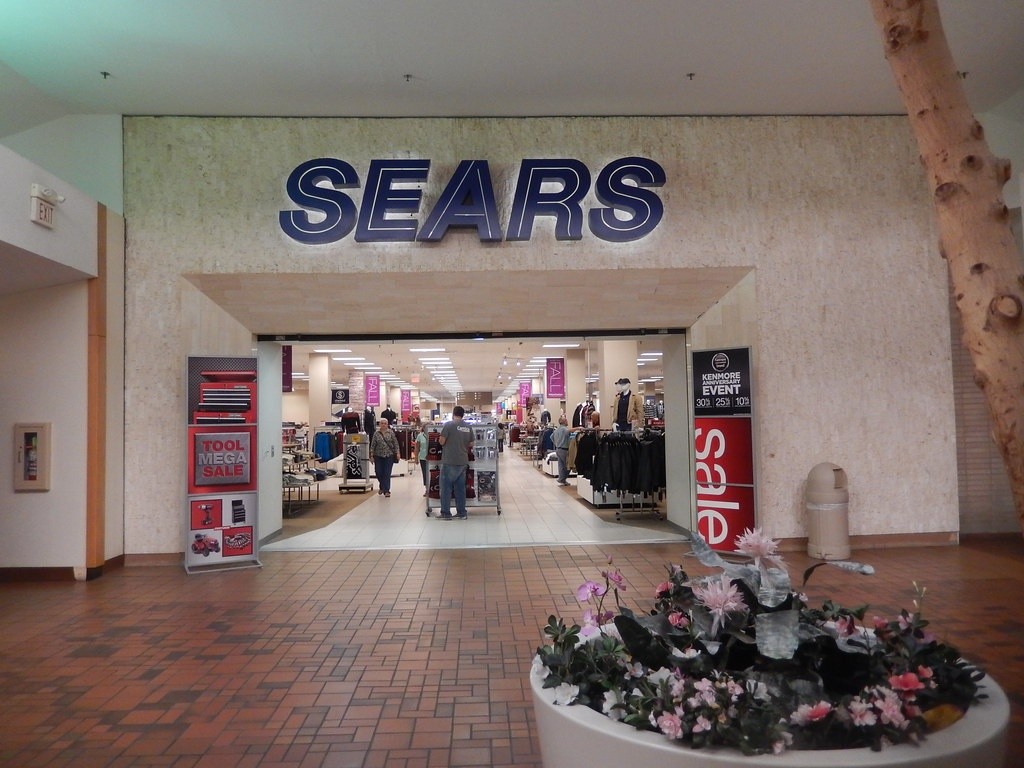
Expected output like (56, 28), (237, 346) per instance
(556, 447), (567, 450)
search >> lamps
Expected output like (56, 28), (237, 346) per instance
(30, 183), (67, 230)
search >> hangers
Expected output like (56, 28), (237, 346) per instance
(602, 432), (632, 446)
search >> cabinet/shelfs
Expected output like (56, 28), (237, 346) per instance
(282, 442), (322, 519)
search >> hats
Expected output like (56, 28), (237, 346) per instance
(615, 378), (630, 384)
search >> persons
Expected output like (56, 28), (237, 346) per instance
(370, 418), (400, 497)
(364, 405), (376, 447)
(414, 422), (429, 497)
(550, 417), (581, 486)
(497, 423), (506, 452)
(341, 407), (361, 434)
(435, 406), (475, 520)
(381, 404), (396, 425)
(572, 400), (600, 428)
(610, 378), (644, 432)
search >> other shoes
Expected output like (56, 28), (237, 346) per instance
(378, 489), (382, 495)
(384, 492), (389, 497)
(452, 514), (467, 520)
(434, 512), (452, 521)
(556, 479), (569, 486)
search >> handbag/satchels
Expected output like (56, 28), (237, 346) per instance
(393, 453), (398, 463)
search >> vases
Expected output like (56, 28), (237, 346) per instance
(529, 622), (1011, 768)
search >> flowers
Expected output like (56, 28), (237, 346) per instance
(536, 527), (991, 752)
